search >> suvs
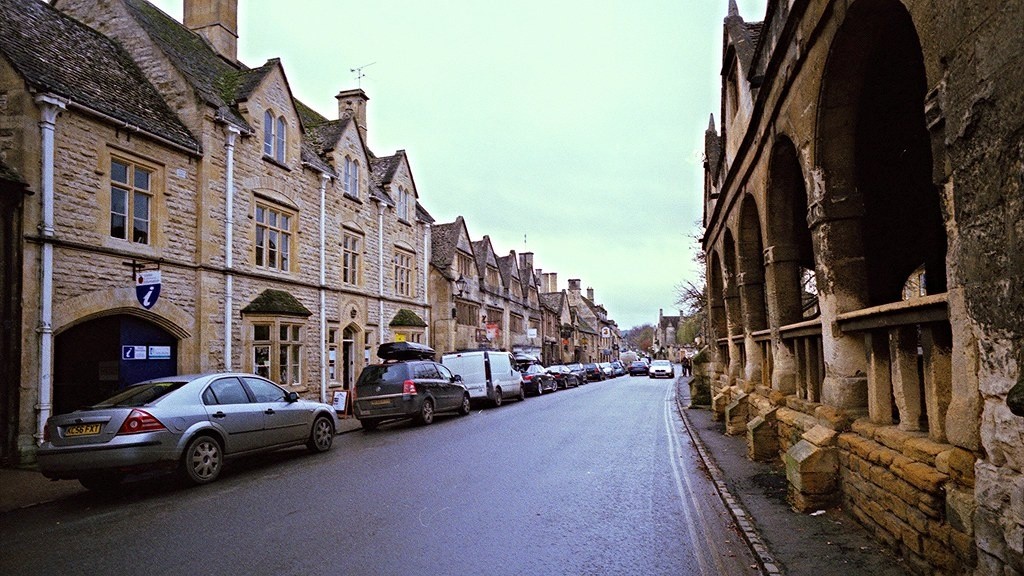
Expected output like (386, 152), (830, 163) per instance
(352, 342), (471, 429)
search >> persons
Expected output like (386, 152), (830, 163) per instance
(680, 356), (692, 377)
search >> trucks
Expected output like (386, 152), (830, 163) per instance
(440, 348), (525, 409)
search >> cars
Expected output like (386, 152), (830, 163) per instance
(38, 371), (340, 489)
(583, 364), (607, 383)
(648, 360), (675, 379)
(628, 361), (652, 377)
(598, 362), (615, 379)
(545, 365), (580, 390)
(517, 364), (558, 396)
(563, 361), (588, 385)
(610, 362), (626, 376)
